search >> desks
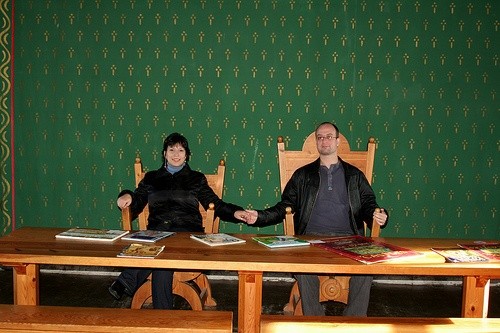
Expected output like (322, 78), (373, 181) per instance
(0, 227), (500, 333)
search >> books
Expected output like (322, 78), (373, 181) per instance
(55, 227), (500, 264)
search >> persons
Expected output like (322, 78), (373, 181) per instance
(241, 122), (389, 317)
(110, 133), (250, 310)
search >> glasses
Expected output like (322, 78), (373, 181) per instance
(316, 136), (337, 140)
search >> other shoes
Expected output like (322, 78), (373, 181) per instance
(109, 280), (125, 299)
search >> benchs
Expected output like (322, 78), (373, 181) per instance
(258, 312), (500, 333)
(0, 304), (235, 333)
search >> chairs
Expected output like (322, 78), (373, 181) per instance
(121, 154), (227, 309)
(278, 130), (383, 315)
